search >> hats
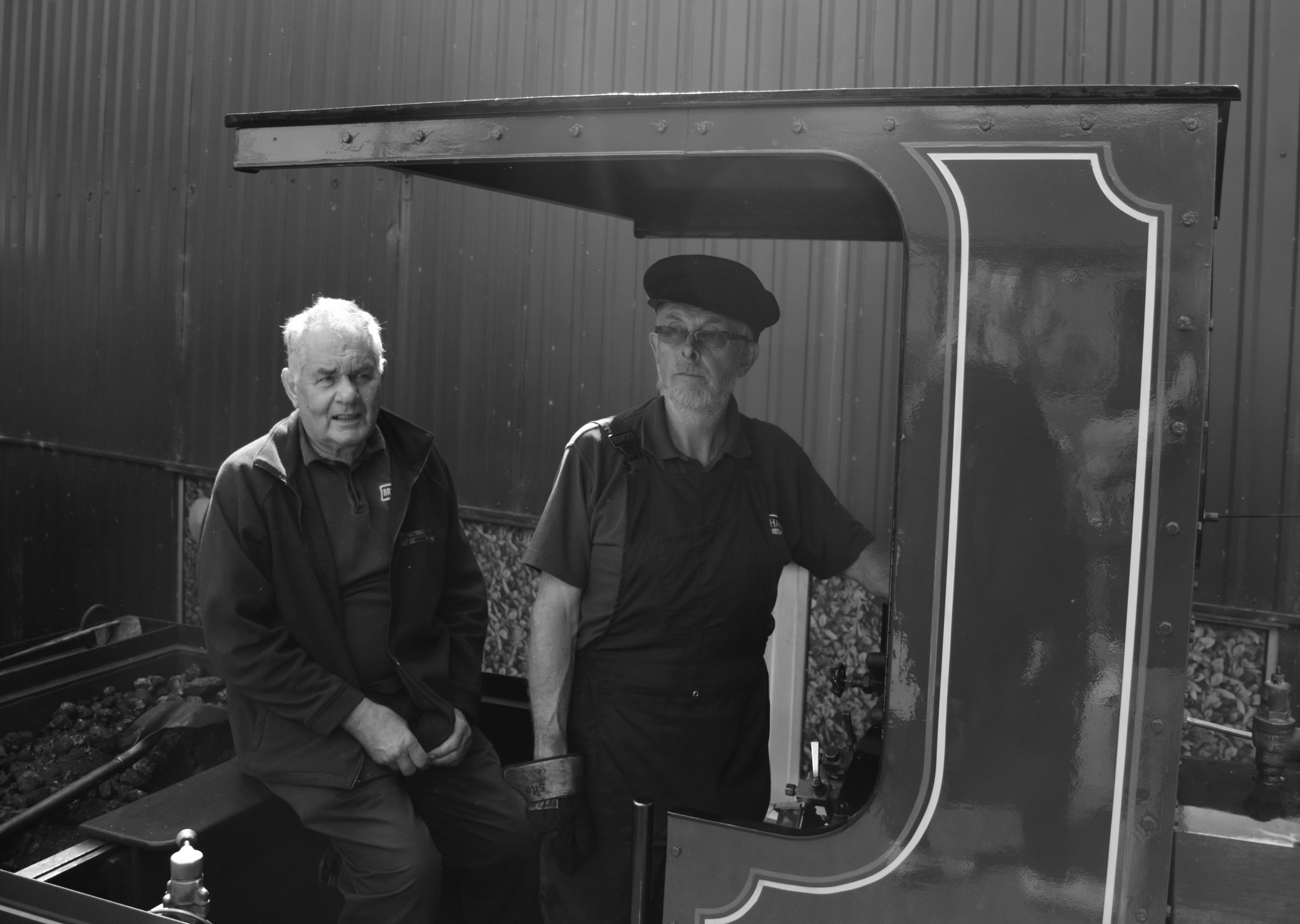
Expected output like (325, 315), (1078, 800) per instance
(643, 254), (780, 332)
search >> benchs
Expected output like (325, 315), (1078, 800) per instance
(71, 710), (312, 862)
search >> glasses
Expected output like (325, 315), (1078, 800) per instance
(653, 324), (750, 350)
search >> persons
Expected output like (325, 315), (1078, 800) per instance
(520, 254), (889, 924)
(195, 297), (533, 924)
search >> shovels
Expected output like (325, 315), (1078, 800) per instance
(0, 695), (230, 842)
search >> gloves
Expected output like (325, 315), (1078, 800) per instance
(504, 754), (599, 873)
